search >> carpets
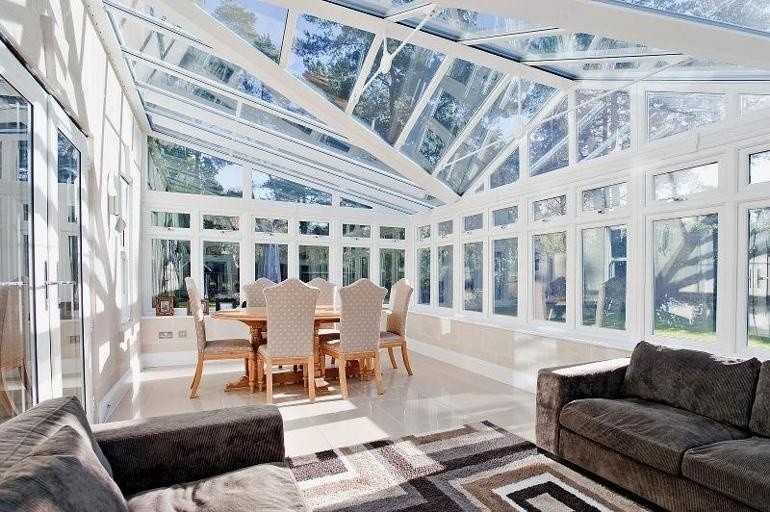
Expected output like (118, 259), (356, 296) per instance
(284, 416), (659, 512)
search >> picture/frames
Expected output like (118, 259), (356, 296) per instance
(186, 299), (209, 316)
(214, 297), (237, 311)
(155, 296), (174, 317)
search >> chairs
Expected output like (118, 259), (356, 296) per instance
(183, 273), (418, 408)
(0, 278), (36, 418)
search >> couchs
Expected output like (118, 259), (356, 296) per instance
(2, 391), (312, 511)
(530, 337), (769, 511)
